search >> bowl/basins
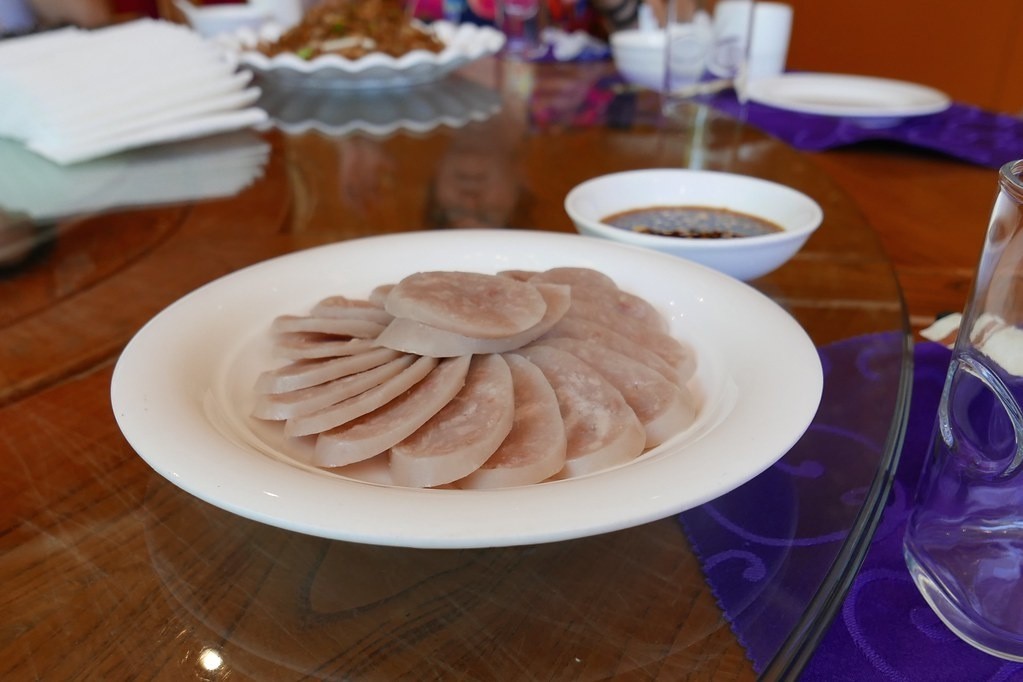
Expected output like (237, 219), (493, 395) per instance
(607, 28), (707, 90)
(564, 168), (823, 287)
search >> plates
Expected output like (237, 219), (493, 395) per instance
(108, 223), (827, 552)
(743, 72), (955, 118)
(204, 20), (512, 92)
(251, 82), (506, 134)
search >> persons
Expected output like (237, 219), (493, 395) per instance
(411, 0), (641, 62)
(424, 55), (633, 231)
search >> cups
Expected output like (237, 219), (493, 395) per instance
(902, 160), (1023, 665)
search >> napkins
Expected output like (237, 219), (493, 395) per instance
(0, 121), (277, 222)
(0, 11), (264, 165)
